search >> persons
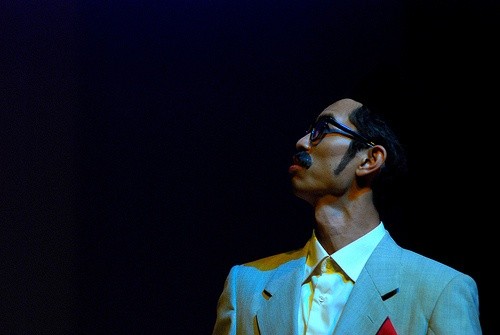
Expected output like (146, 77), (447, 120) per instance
(209, 97), (483, 334)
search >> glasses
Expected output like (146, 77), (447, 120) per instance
(306, 118), (376, 148)
